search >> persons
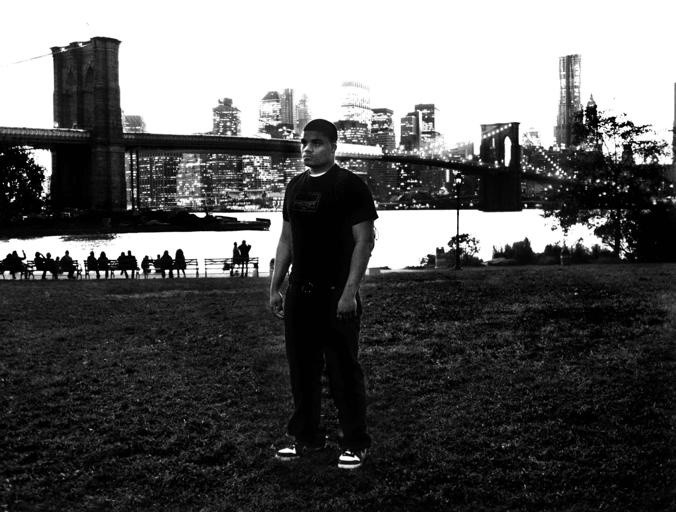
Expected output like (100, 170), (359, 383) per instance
(230, 268), (239, 277)
(269, 258), (274, 277)
(237, 240), (251, 269)
(230, 242), (240, 269)
(268, 119), (380, 468)
(1, 249), (188, 279)
(240, 267), (249, 277)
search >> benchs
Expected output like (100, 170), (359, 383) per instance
(0, 257), (260, 280)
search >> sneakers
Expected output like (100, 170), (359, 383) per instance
(276, 441), (326, 461)
(338, 448), (368, 470)
